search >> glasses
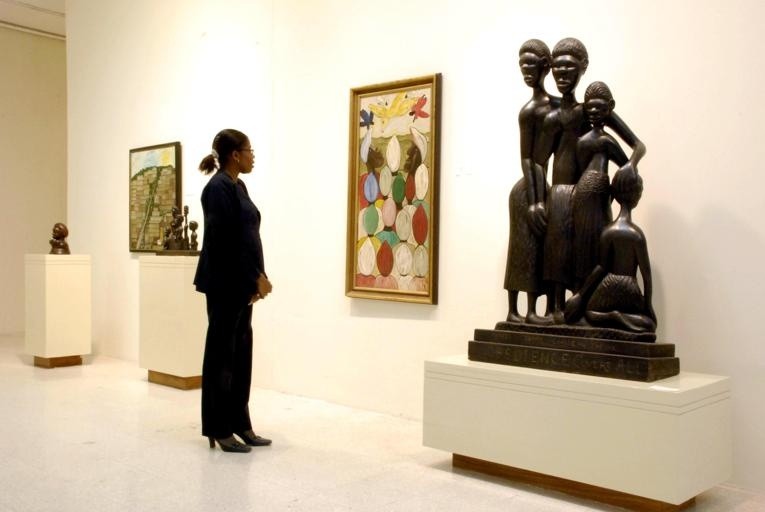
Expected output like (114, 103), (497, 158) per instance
(237, 148), (255, 153)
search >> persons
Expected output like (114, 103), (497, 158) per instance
(163, 205), (198, 251)
(505, 37), (657, 333)
(49, 223), (70, 254)
(193, 129), (272, 454)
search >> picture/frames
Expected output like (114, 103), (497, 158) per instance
(344, 73), (442, 304)
(128, 141), (182, 253)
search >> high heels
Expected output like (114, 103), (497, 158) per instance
(210, 430), (272, 452)
